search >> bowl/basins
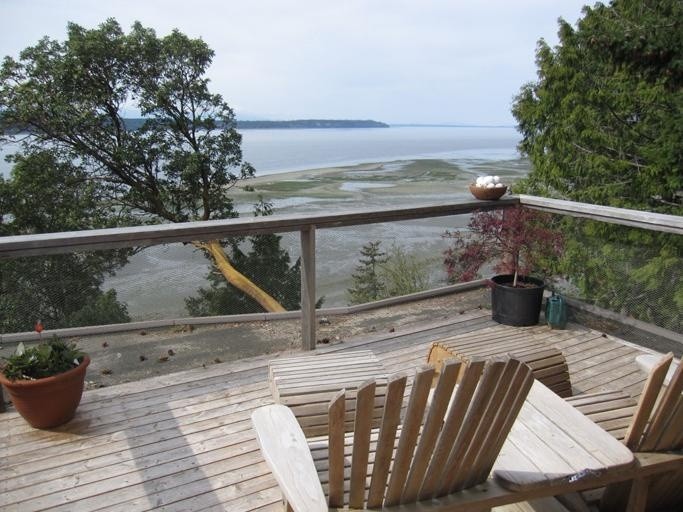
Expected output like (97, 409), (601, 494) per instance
(470, 185), (507, 200)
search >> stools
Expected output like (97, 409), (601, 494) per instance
(266, 350), (402, 437)
(427, 328), (573, 398)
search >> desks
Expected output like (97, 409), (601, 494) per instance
(425, 377), (635, 512)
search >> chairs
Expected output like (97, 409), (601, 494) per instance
(252, 358), (536, 512)
(562, 352), (683, 512)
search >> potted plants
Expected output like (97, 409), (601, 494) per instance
(0, 319), (90, 429)
(441, 204), (565, 327)
